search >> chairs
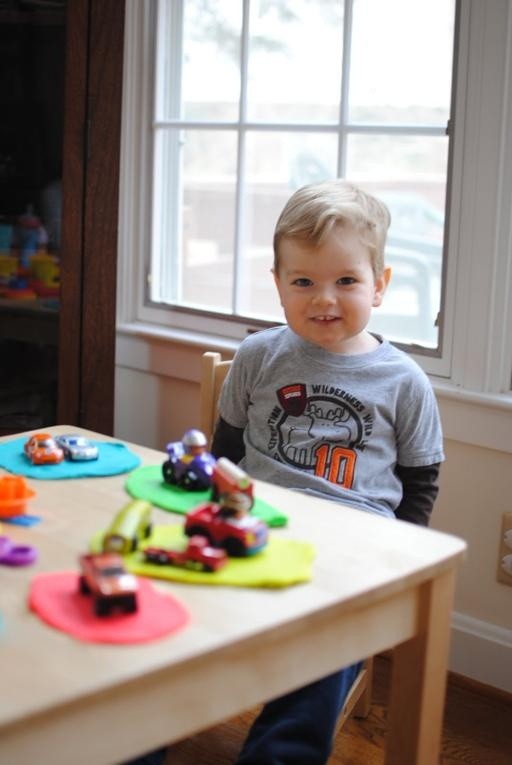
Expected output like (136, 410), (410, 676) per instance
(190, 345), (412, 744)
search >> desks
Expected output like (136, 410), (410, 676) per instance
(1, 292), (60, 360)
(2, 422), (470, 763)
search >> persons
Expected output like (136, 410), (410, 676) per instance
(131, 182), (446, 765)
